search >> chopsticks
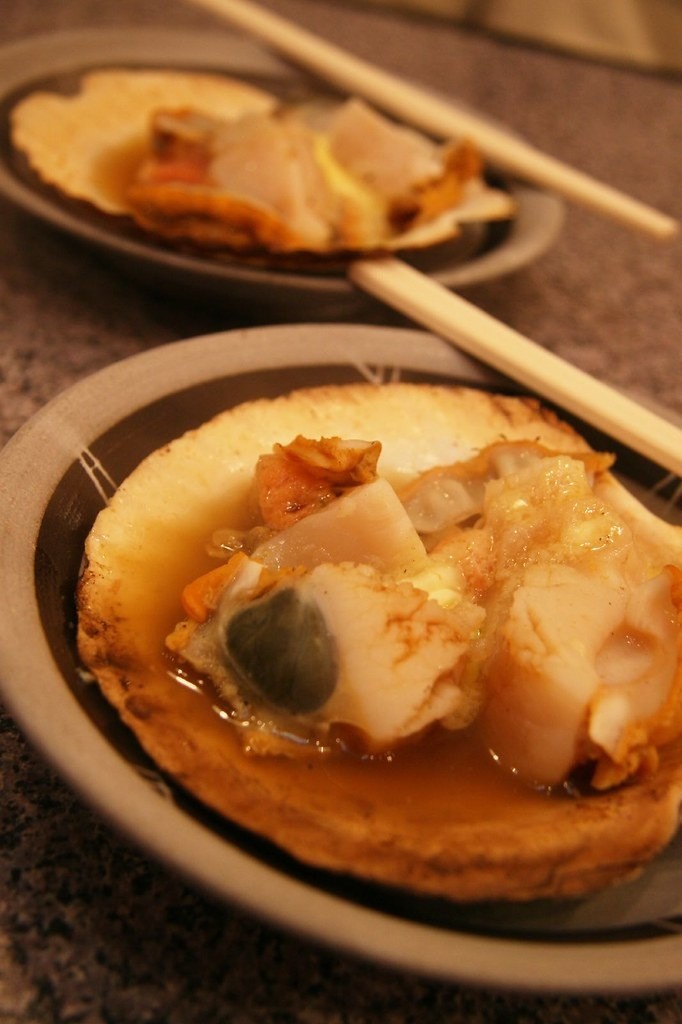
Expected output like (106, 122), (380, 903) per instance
(191, 0), (678, 241)
(347, 253), (682, 479)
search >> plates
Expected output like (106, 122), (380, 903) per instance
(0, 314), (682, 997)
(0, 24), (564, 292)
